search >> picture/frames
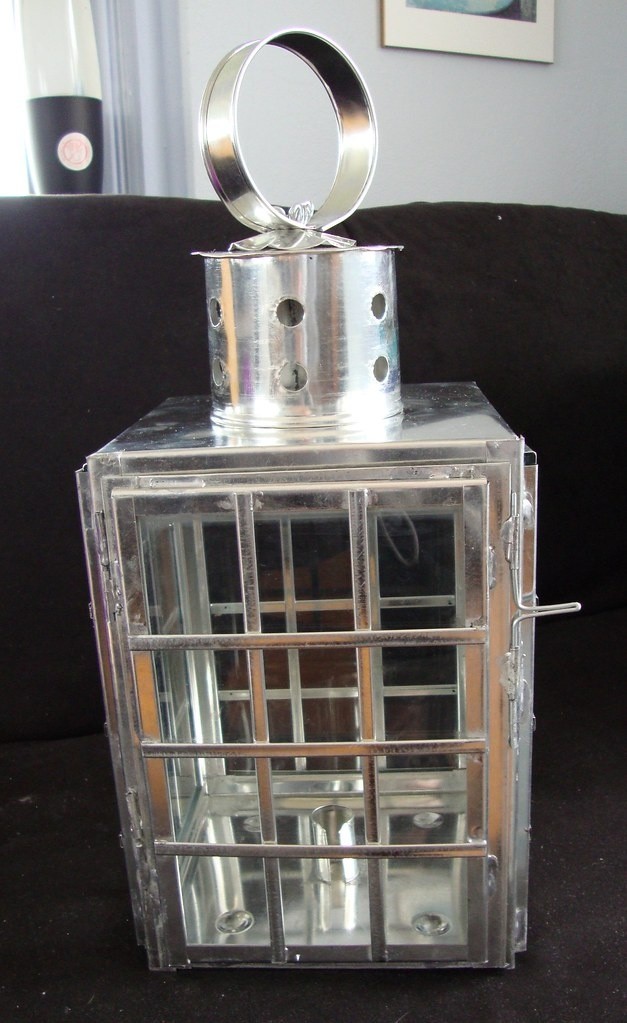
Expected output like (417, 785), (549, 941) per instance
(380, 0), (558, 68)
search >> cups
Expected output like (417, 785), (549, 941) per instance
(26, 95), (107, 197)
